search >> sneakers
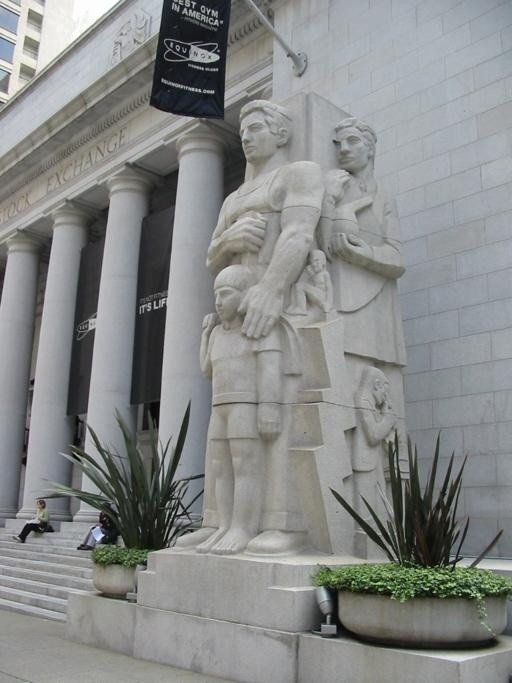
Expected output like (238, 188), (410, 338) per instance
(80, 544), (92, 550)
(77, 544), (85, 550)
(12, 535), (22, 542)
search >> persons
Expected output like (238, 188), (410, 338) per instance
(350, 363), (396, 529)
(288, 245), (335, 319)
(77, 502), (120, 550)
(190, 265), (286, 556)
(328, 169), (380, 247)
(14, 497), (56, 547)
(200, 99), (326, 552)
(322, 117), (412, 546)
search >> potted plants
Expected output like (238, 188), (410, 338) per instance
(36, 397), (204, 598)
(309, 428), (512, 649)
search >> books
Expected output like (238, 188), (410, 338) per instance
(91, 525), (105, 541)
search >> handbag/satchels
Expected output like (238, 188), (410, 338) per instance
(100, 528), (118, 543)
(44, 525), (54, 532)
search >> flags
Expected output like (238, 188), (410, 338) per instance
(149, 0), (231, 120)
